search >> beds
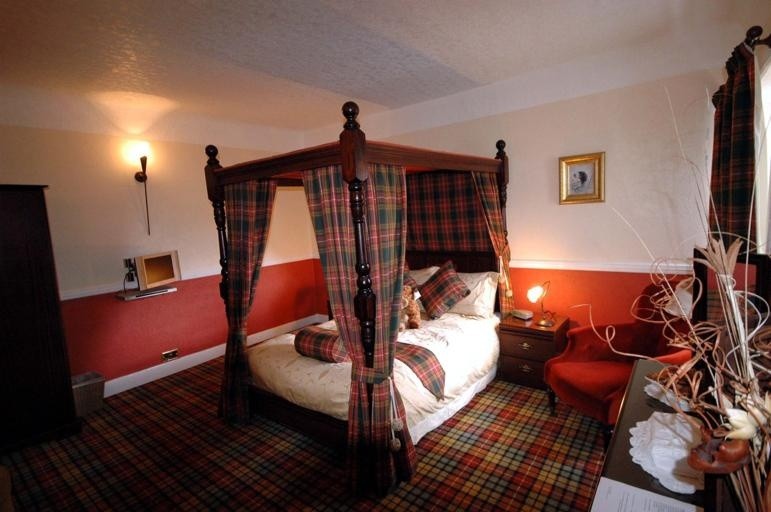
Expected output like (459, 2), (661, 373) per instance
(204, 100), (512, 498)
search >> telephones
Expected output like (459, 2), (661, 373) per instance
(511, 309), (534, 320)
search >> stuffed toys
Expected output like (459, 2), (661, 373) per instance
(399, 285), (420, 330)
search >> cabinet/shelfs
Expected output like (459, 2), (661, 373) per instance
(0, 182), (77, 456)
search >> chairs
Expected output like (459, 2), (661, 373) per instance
(543, 279), (694, 455)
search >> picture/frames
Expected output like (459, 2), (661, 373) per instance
(558, 150), (606, 206)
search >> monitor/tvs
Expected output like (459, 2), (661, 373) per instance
(134, 250), (181, 292)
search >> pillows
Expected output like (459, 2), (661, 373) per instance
(406, 257), (501, 322)
(294, 322), (353, 364)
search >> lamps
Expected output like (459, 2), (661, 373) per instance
(527, 278), (559, 328)
(133, 137), (151, 237)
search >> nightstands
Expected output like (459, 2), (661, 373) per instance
(495, 311), (571, 392)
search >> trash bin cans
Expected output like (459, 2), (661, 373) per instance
(70, 371), (106, 417)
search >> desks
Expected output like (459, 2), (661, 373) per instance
(588, 358), (724, 512)
